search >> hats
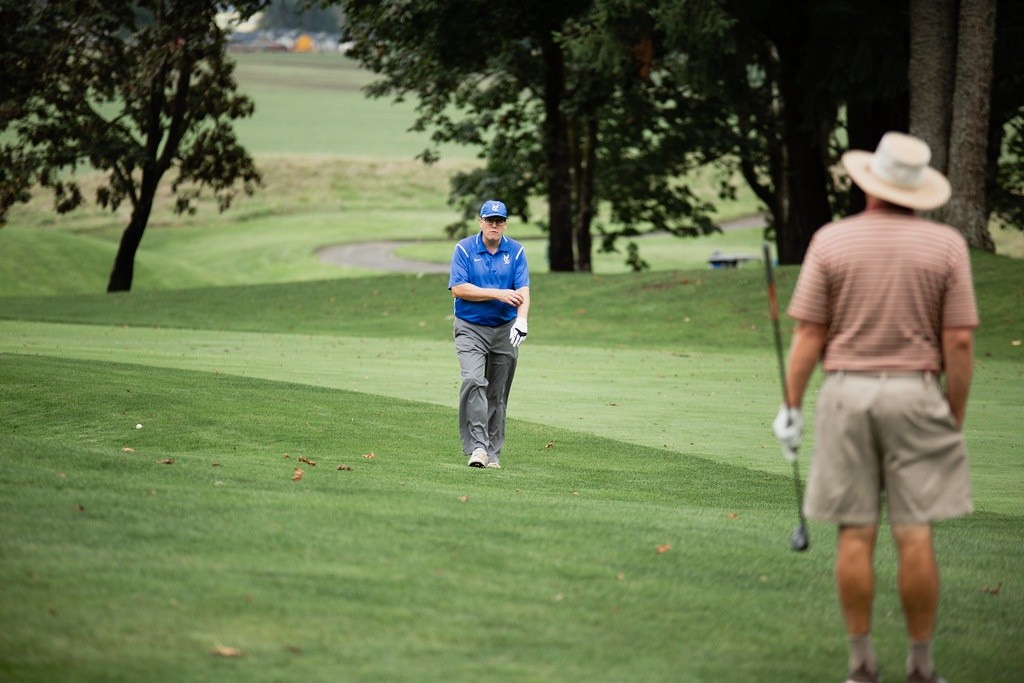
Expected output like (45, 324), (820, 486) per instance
(480, 200), (507, 218)
(843, 131), (951, 210)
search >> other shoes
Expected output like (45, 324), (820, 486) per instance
(846, 664), (878, 683)
(908, 668), (936, 683)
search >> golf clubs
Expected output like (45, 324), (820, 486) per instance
(763, 243), (809, 555)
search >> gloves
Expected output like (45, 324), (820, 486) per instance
(509, 318), (527, 348)
(773, 405), (805, 464)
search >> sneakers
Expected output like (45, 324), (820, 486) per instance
(467, 449), (488, 467)
(487, 462), (501, 468)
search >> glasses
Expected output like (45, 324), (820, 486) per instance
(483, 219), (505, 226)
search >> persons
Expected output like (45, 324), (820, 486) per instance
(773, 132), (981, 683)
(448, 201), (530, 468)
